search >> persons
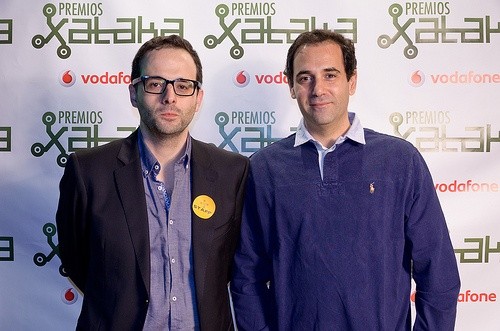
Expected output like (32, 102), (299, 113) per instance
(230, 29), (461, 331)
(56, 34), (249, 331)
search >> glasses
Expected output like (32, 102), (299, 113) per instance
(132, 75), (202, 96)
